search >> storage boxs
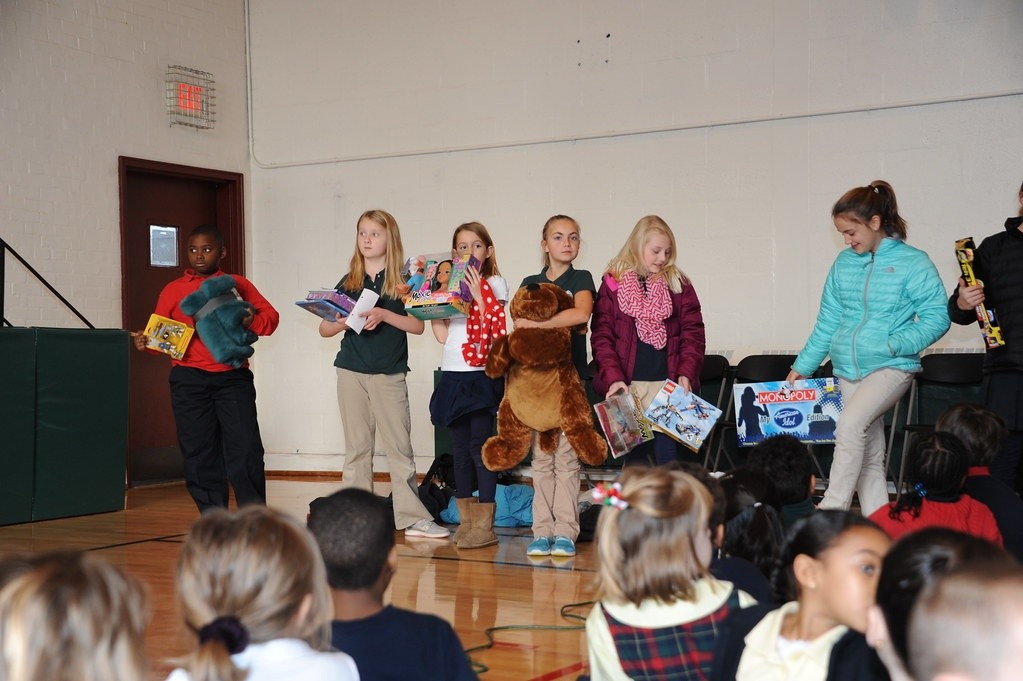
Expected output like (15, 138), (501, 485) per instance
(955, 237), (1006, 350)
(605, 385), (655, 452)
(401, 253), (481, 321)
(732, 378), (843, 447)
(645, 378), (724, 452)
(306, 292), (355, 312)
(142, 314), (195, 361)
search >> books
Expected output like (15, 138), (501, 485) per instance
(141, 312), (196, 362)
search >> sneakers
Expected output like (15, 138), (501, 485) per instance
(405, 517), (450, 538)
(527, 536), (576, 556)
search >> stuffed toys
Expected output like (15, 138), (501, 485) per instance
(179, 273), (258, 366)
(481, 284), (607, 471)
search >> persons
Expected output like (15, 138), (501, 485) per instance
(948, 184), (1023, 492)
(321, 210), (427, 537)
(126, 223), (280, 514)
(0, 404), (1022, 680)
(791, 182), (952, 518)
(405, 257), (454, 295)
(421, 214), (706, 558)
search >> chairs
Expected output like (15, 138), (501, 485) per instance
(496, 353), (987, 518)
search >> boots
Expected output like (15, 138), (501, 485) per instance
(453, 496), (499, 548)
